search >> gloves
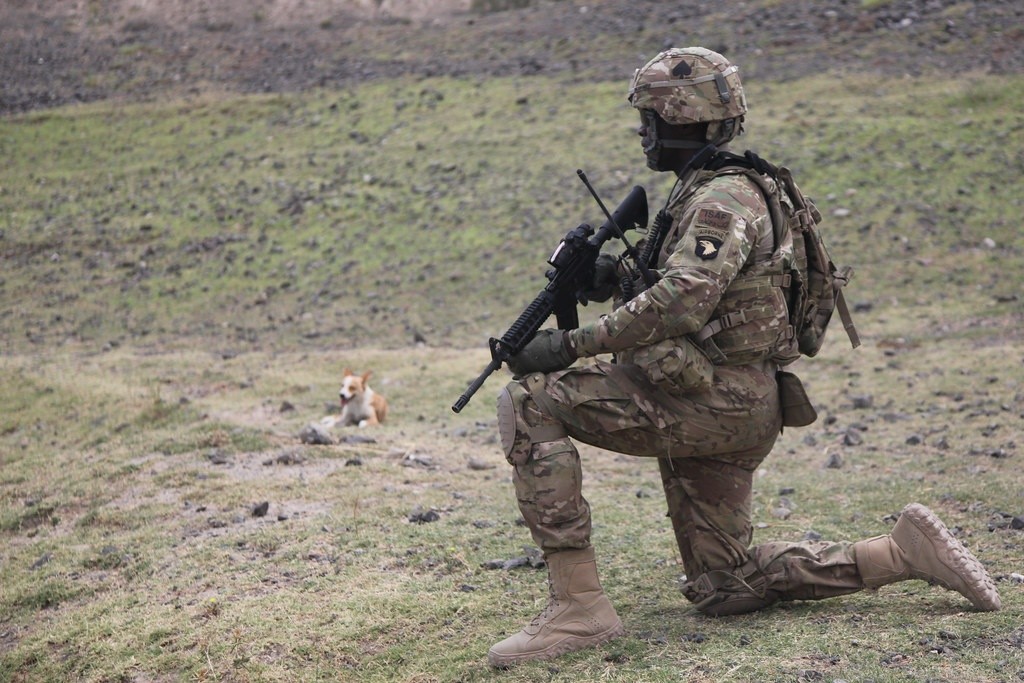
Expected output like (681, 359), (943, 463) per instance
(506, 328), (574, 380)
(575, 253), (620, 305)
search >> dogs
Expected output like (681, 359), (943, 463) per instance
(321, 367), (386, 430)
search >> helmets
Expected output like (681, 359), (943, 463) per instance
(627, 47), (747, 125)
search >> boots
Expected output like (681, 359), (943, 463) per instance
(488, 545), (624, 669)
(854, 503), (1002, 611)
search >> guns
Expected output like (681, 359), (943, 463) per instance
(453, 186), (649, 412)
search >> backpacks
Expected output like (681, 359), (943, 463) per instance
(680, 165), (861, 358)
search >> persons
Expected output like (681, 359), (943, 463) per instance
(485, 45), (1003, 669)
